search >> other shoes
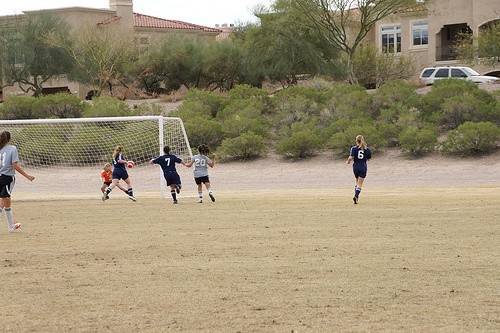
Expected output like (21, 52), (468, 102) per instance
(106, 195), (109, 199)
(197, 198), (203, 203)
(174, 184), (180, 194)
(172, 199), (177, 204)
(102, 192), (107, 201)
(353, 195), (358, 204)
(9, 223), (22, 233)
(129, 195), (136, 201)
(209, 192), (215, 202)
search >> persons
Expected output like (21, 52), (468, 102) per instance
(101, 146), (137, 201)
(346, 135), (370, 204)
(100, 163), (132, 199)
(0, 130), (36, 233)
(186, 145), (216, 203)
(149, 146), (191, 204)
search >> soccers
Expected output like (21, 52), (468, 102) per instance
(127, 160), (135, 168)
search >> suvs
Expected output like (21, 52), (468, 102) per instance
(419, 65), (500, 86)
